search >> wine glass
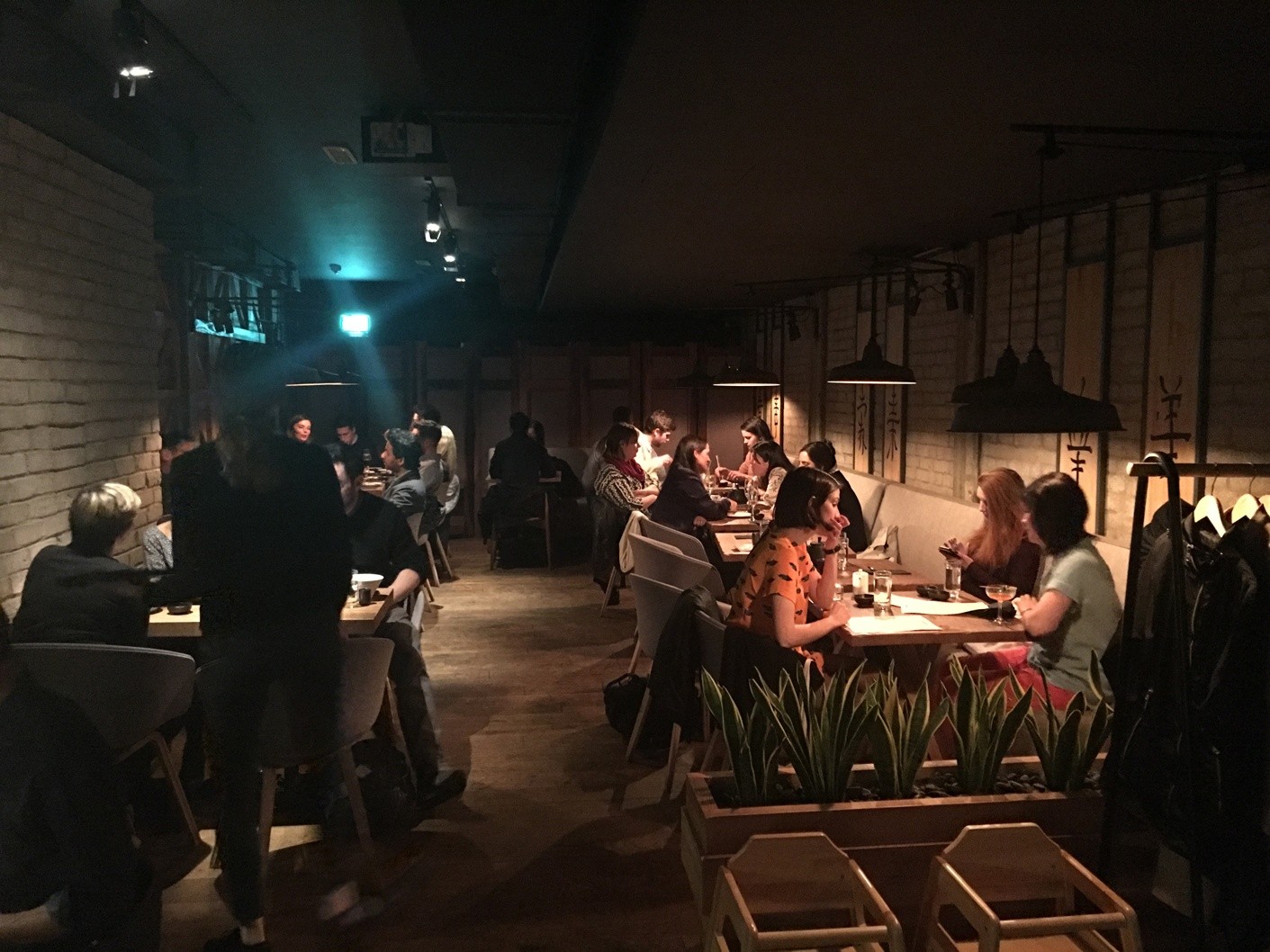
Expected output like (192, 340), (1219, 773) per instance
(985, 584), (1017, 626)
(744, 477), (759, 522)
(702, 475), (715, 500)
(362, 448), (372, 474)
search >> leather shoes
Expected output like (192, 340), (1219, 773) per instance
(205, 928), (269, 952)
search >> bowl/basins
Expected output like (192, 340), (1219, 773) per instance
(927, 590), (950, 600)
(916, 584), (938, 598)
(351, 573), (385, 600)
(854, 593), (874, 606)
(167, 602), (192, 614)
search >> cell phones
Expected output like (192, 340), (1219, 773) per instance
(940, 545), (960, 560)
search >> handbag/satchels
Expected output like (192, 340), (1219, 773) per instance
(604, 674), (684, 752)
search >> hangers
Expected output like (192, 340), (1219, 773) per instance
(1257, 494), (1270, 519)
(1230, 458), (1260, 524)
(1192, 461), (1228, 540)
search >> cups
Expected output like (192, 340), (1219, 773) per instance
(837, 538), (849, 571)
(945, 557), (963, 601)
(834, 583), (843, 602)
(839, 530), (846, 543)
(752, 532), (760, 546)
(872, 570), (893, 612)
(359, 588), (371, 606)
(718, 475), (728, 487)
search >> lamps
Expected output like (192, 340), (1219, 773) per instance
(946, 168), (1270, 405)
(901, 266), (959, 318)
(711, 268), (934, 388)
(824, 248), (978, 388)
(423, 170), (468, 283)
(786, 304), (802, 343)
(114, 0), (154, 81)
(945, 116), (1270, 436)
(672, 304), (820, 390)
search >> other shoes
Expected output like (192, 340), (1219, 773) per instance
(437, 770), (465, 806)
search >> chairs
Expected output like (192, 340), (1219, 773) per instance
(702, 829), (908, 952)
(912, 820), (1144, 952)
(0, 449), (463, 866)
(483, 435), (1138, 794)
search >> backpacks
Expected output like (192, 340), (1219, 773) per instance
(115, 733), (216, 892)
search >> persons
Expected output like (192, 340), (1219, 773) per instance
(726, 465), (856, 756)
(8, 478), (197, 822)
(162, 370), (375, 942)
(947, 465), (1043, 610)
(925, 471), (1123, 756)
(135, 387), (872, 625)
(314, 443), (465, 809)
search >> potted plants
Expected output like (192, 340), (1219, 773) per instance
(676, 646), (1115, 932)
